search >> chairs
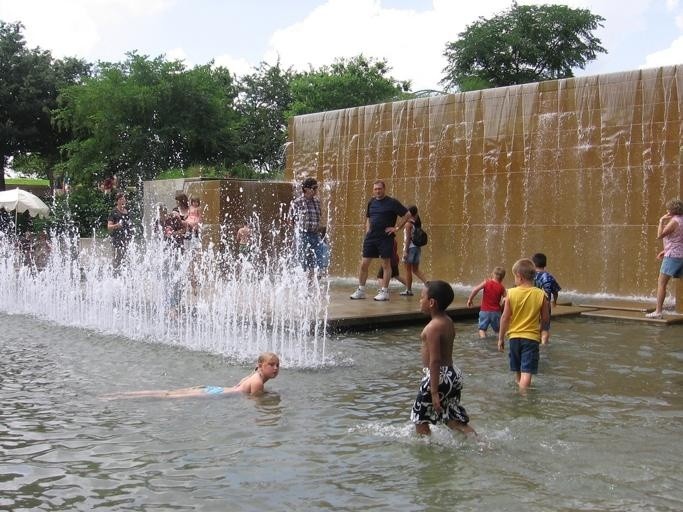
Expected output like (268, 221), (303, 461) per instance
(645, 311), (663, 319)
(374, 292), (389, 300)
(350, 289), (366, 299)
(400, 290), (412, 295)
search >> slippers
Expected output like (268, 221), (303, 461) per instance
(411, 221), (427, 246)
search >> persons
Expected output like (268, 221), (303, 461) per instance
(497, 260), (549, 393)
(350, 181), (409, 300)
(529, 253), (561, 343)
(408, 280), (477, 437)
(152, 191), (207, 320)
(644, 198), (682, 318)
(94, 353), (278, 402)
(377, 232), (407, 292)
(235, 219), (253, 262)
(287, 178), (329, 296)
(101, 176), (117, 196)
(466, 266), (507, 338)
(50, 172), (71, 221)
(400, 205), (429, 295)
(108, 192), (130, 277)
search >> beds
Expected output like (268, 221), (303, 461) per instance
(0, 186), (48, 238)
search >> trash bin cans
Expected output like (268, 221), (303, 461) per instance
(394, 225), (399, 231)
